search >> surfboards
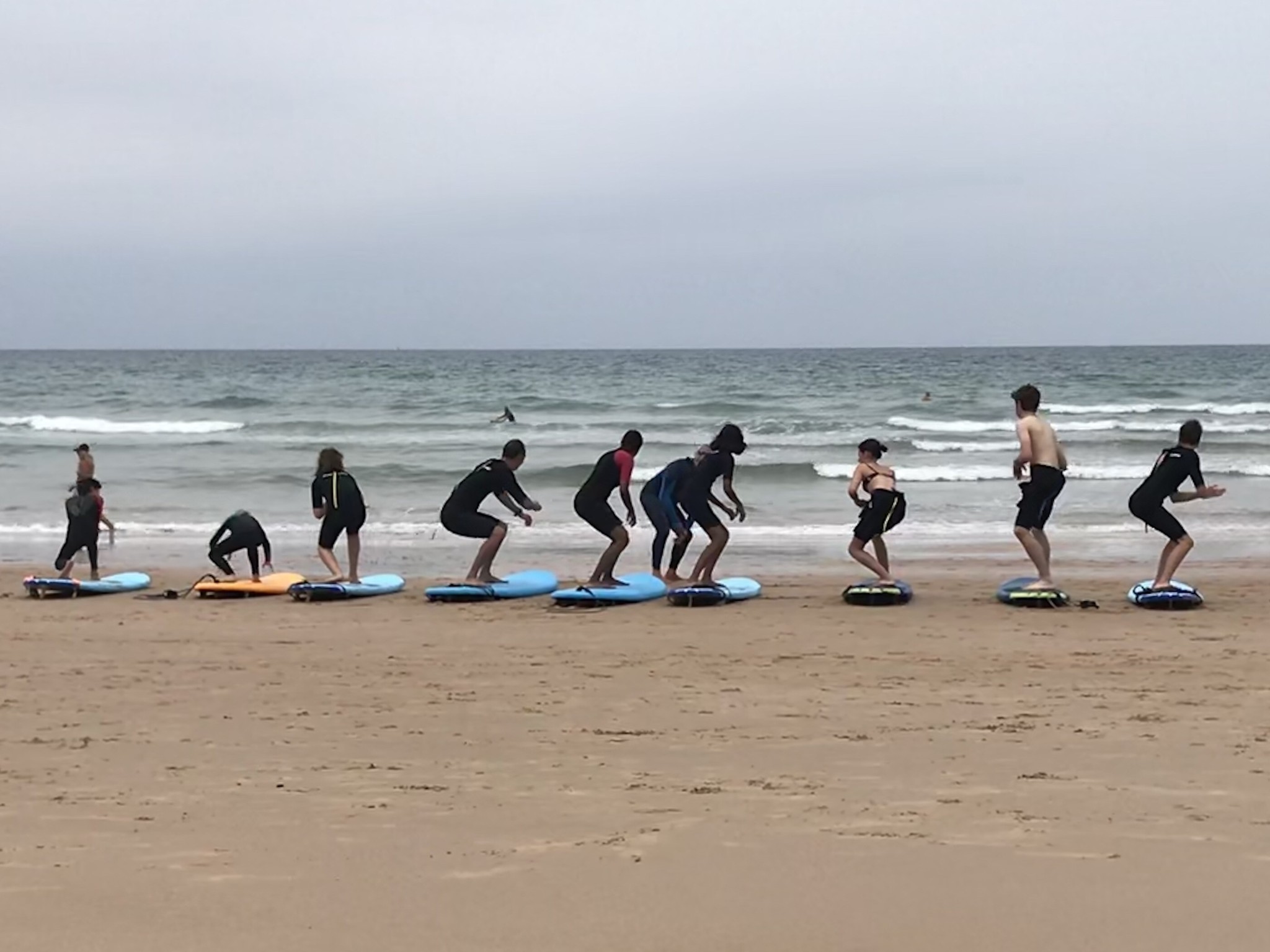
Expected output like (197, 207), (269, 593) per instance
(842, 578), (912, 607)
(997, 577), (1071, 609)
(668, 577), (761, 606)
(424, 568), (558, 603)
(23, 572), (151, 600)
(1127, 579), (1204, 610)
(195, 572), (306, 597)
(289, 573), (405, 602)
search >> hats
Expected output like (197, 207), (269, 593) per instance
(74, 444), (89, 451)
(710, 425), (745, 455)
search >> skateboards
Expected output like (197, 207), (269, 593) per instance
(551, 573), (667, 608)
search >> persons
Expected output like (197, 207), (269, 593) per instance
(311, 447), (366, 583)
(55, 477), (104, 581)
(1128, 419), (1226, 592)
(1011, 383), (1068, 590)
(849, 438), (907, 585)
(639, 443), (717, 584)
(68, 444), (114, 529)
(681, 424), (747, 586)
(440, 438), (542, 587)
(574, 429), (643, 589)
(208, 509), (274, 583)
(496, 406), (515, 423)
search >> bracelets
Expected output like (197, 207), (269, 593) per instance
(1013, 459), (1027, 471)
(513, 509), (522, 516)
